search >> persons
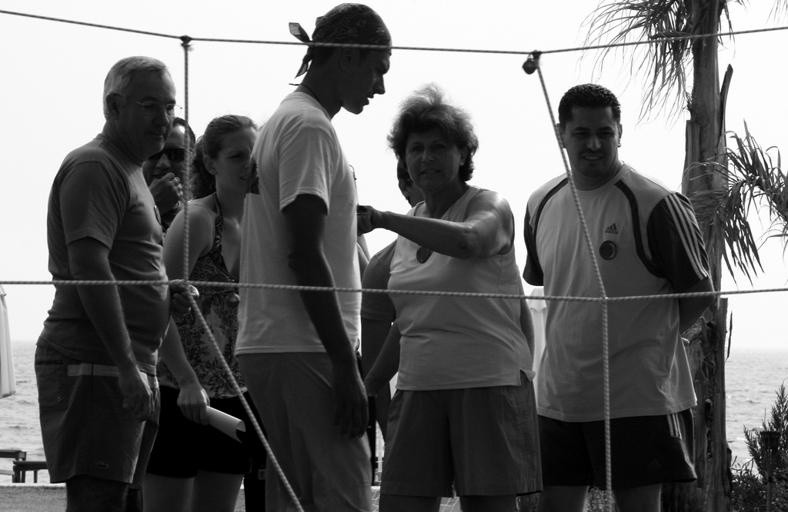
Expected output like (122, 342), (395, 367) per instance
(356, 86), (544, 512)
(144, 116), (195, 225)
(523, 84), (715, 511)
(33, 55), (179, 511)
(345, 163), (370, 282)
(141, 114), (260, 512)
(233, 2), (393, 512)
(359, 237), (535, 442)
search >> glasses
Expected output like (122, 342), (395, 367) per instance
(146, 145), (185, 163)
(115, 94), (184, 121)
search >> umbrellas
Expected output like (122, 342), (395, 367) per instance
(0, 284), (19, 399)
